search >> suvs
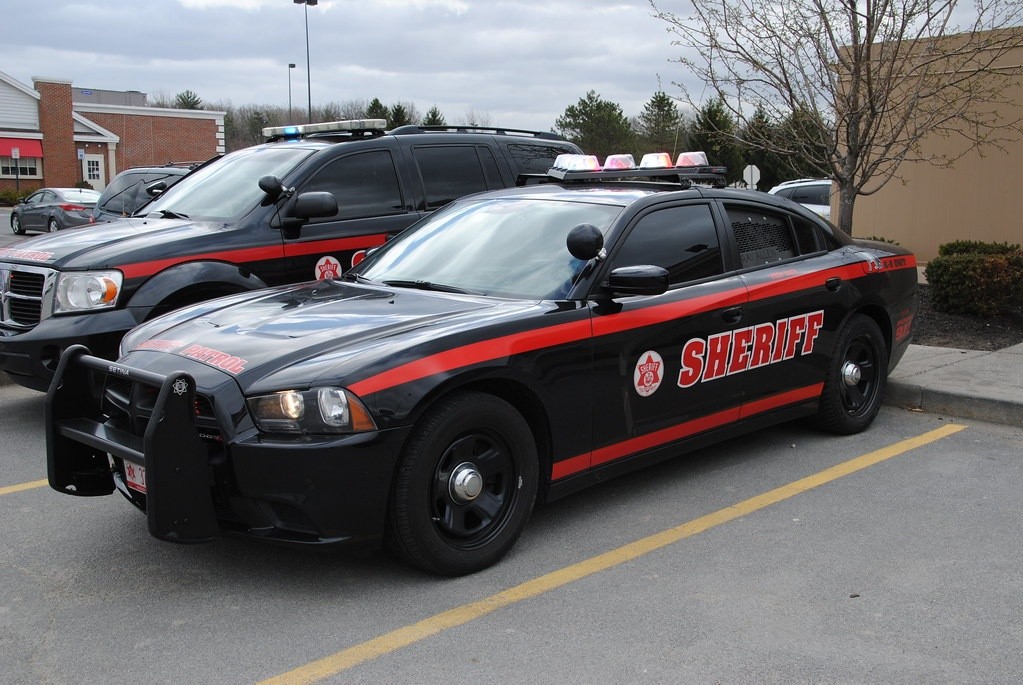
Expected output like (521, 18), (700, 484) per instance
(88, 159), (206, 225)
(0, 117), (602, 396)
(766, 175), (832, 224)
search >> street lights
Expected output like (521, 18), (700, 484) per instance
(288, 63), (296, 126)
(733, 79), (746, 139)
(293, 0), (318, 124)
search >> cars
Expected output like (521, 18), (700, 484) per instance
(42, 150), (921, 583)
(9, 187), (103, 236)
